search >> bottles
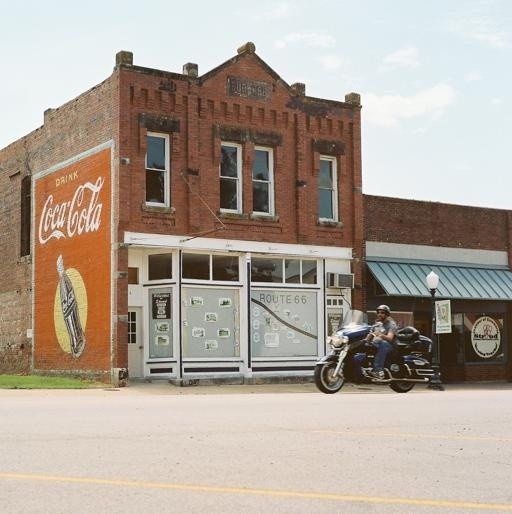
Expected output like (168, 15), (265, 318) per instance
(57, 253), (86, 359)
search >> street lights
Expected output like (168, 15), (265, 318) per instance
(426, 271), (443, 390)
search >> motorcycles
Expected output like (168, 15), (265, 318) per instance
(313, 308), (436, 393)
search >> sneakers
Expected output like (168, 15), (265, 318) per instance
(366, 369), (386, 379)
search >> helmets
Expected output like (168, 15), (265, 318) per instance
(376, 304), (391, 314)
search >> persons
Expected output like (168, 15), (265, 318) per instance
(365, 304), (398, 379)
